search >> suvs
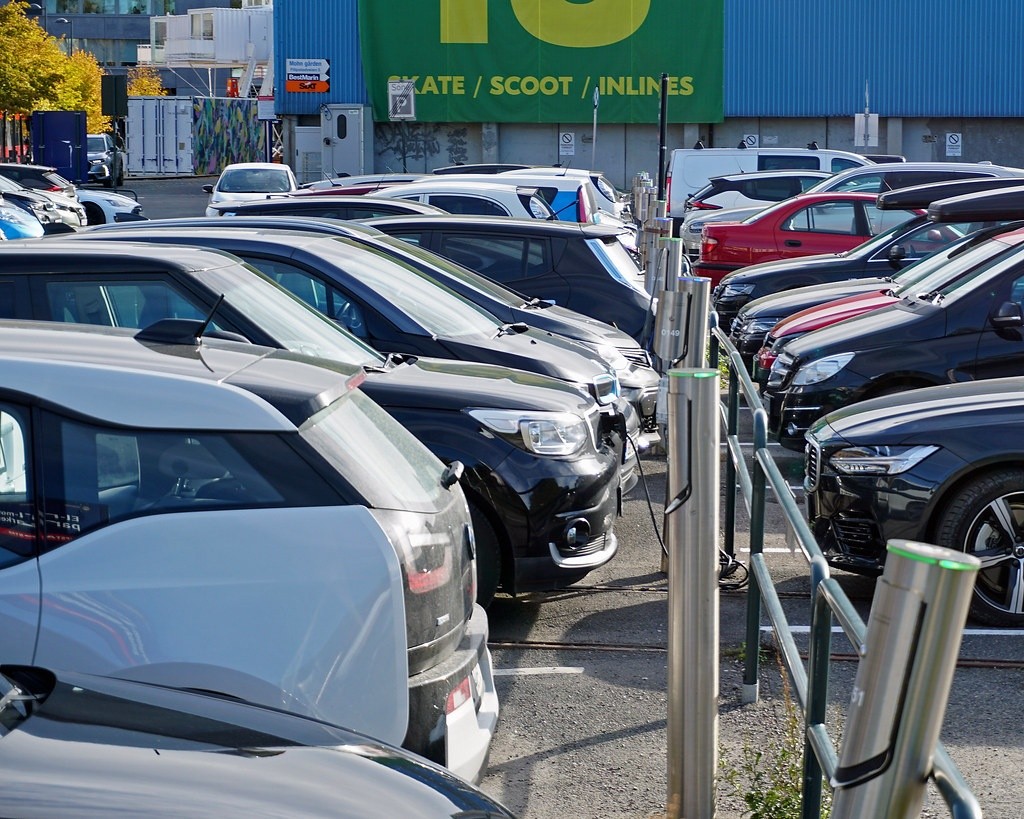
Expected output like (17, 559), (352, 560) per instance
(0, 216), (661, 819)
(86, 134), (126, 189)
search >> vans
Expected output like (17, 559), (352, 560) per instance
(668, 142), (879, 217)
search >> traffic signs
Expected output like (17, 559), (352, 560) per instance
(286, 58), (331, 93)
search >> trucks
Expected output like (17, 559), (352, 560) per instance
(24, 110), (87, 189)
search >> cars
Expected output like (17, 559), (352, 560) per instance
(0, 161), (153, 240)
(679, 154), (1024, 619)
(211, 161), (631, 219)
(200, 162), (297, 219)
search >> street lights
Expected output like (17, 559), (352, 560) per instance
(31, 2), (47, 31)
(54, 16), (74, 60)
